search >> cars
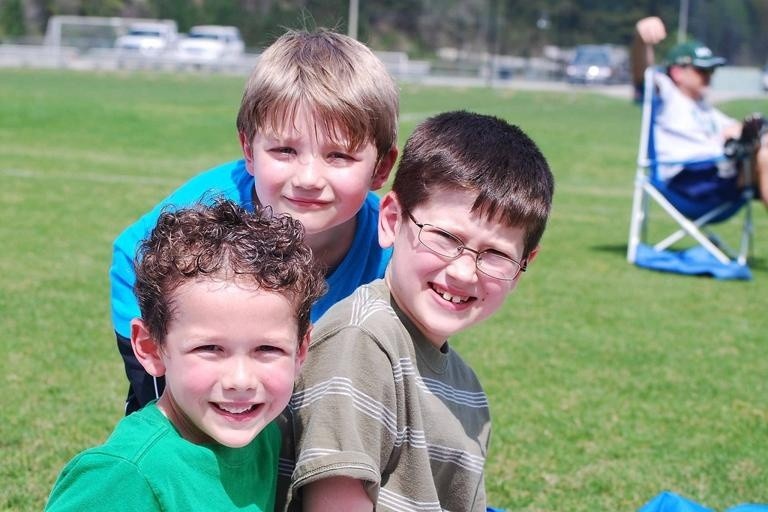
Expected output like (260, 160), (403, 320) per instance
(118, 23), (246, 55)
(566, 46), (630, 83)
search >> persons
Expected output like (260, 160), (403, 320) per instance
(43, 187), (330, 512)
(271, 108), (554, 512)
(631, 16), (768, 209)
(107, 26), (399, 417)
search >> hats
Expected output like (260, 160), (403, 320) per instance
(664, 41), (728, 69)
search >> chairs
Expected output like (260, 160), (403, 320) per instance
(625, 65), (753, 281)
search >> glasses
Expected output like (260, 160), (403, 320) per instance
(401, 203), (529, 282)
